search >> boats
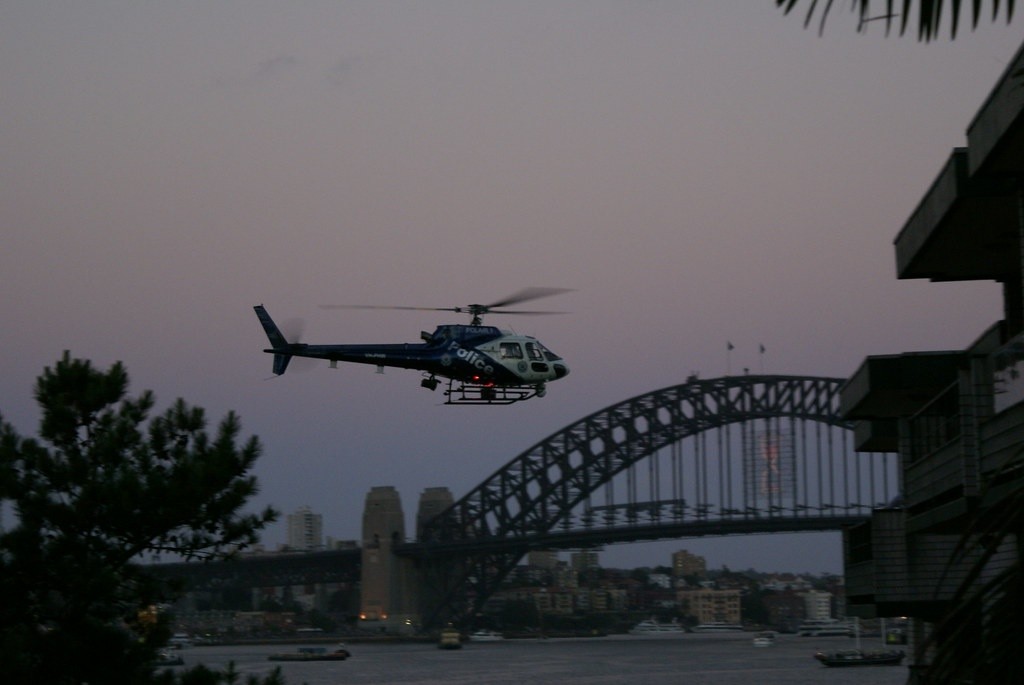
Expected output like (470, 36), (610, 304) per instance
(813, 649), (907, 668)
(797, 618), (850, 637)
(573, 625), (608, 636)
(467, 629), (505, 642)
(268, 651), (346, 662)
(437, 628), (462, 650)
(168, 633), (193, 645)
(627, 618), (686, 634)
(695, 621), (743, 632)
(753, 631), (776, 647)
(156, 652), (184, 666)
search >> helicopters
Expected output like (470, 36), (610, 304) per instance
(252, 287), (582, 406)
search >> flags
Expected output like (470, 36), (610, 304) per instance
(728, 342), (735, 350)
(760, 344), (765, 353)
